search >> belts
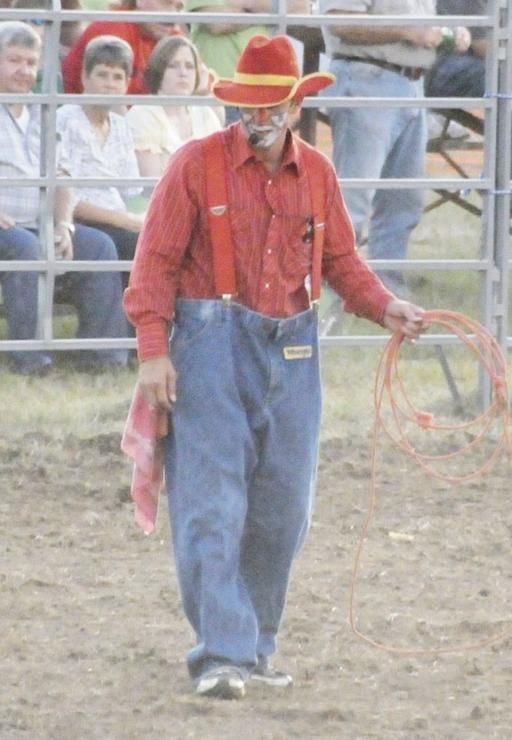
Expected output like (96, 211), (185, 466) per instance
(332, 51), (423, 81)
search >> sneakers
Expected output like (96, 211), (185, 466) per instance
(250, 666), (293, 688)
(384, 278), (410, 300)
(76, 358), (125, 376)
(19, 351), (51, 374)
(197, 671), (245, 700)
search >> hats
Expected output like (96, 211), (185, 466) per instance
(213, 36), (335, 109)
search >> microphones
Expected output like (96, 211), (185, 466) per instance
(238, 110), (259, 144)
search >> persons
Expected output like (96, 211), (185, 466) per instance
(1, 1), (509, 377)
(120, 38), (431, 699)
